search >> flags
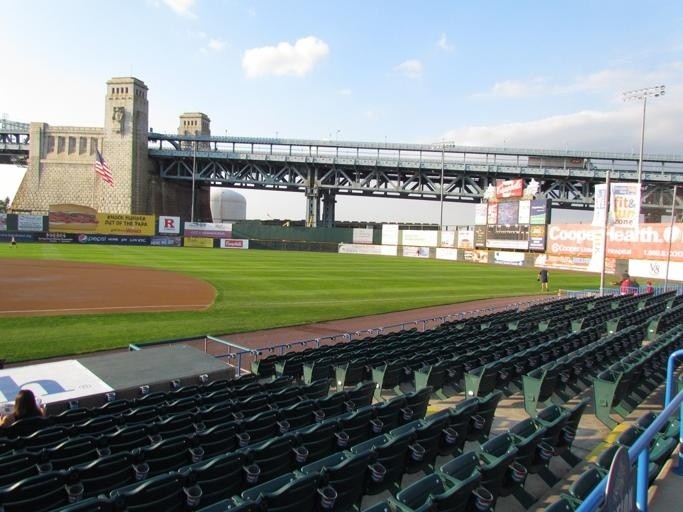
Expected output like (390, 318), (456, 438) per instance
(95, 149), (113, 186)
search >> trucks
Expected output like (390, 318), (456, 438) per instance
(526, 151), (596, 170)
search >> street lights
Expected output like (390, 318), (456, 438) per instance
(618, 83), (666, 185)
(428, 136), (456, 226)
(179, 141), (212, 221)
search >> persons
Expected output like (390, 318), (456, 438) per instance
(619, 269), (654, 295)
(0, 389), (47, 429)
(9, 235), (19, 249)
(538, 266), (548, 292)
